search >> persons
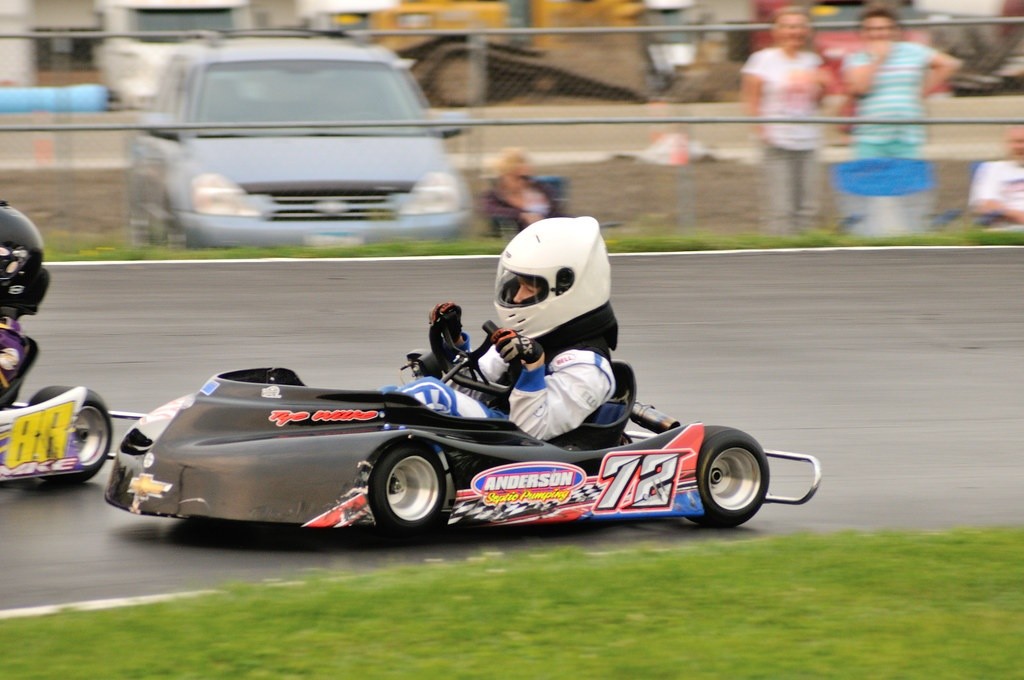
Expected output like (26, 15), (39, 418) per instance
(483, 148), (566, 230)
(381, 216), (618, 441)
(0, 199), (43, 397)
(841, 10), (964, 157)
(968, 126), (1024, 231)
(739, 5), (835, 233)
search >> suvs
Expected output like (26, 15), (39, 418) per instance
(121, 27), (470, 248)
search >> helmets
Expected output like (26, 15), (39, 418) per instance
(0, 200), (44, 315)
(493, 216), (619, 353)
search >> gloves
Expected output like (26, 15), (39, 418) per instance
(428, 302), (463, 343)
(490, 328), (544, 364)
